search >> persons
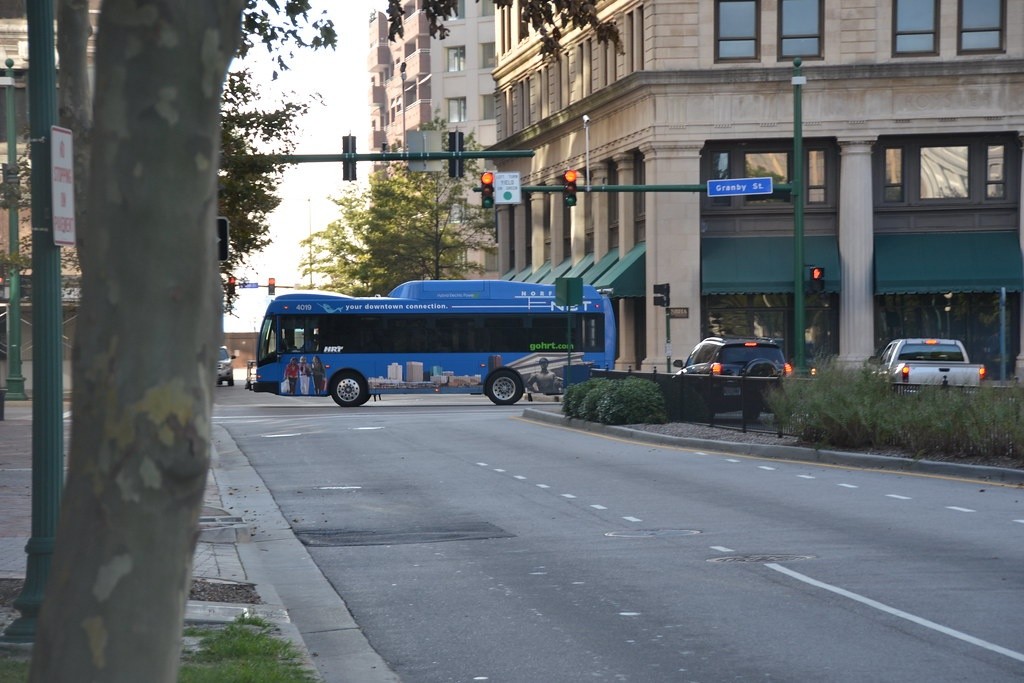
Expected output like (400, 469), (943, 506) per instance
(284, 354), (326, 395)
(526, 358), (564, 394)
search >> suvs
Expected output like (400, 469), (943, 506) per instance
(672, 336), (792, 423)
(216, 345), (236, 386)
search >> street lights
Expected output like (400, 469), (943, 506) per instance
(2, 56), (31, 400)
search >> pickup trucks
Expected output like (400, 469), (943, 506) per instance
(865, 337), (986, 396)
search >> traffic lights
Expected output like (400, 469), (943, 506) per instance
(481, 172), (494, 209)
(563, 170), (577, 207)
(269, 278), (275, 295)
(229, 277), (235, 294)
(809, 265), (826, 293)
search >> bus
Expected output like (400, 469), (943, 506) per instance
(250, 280), (617, 408)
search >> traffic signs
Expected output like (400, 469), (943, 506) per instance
(707, 177), (773, 197)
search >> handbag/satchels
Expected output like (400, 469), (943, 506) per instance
(281, 378), (290, 393)
(320, 378), (327, 391)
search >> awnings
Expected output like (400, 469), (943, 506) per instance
(591, 240), (645, 299)
(563, 252), (594, 278)
(524, 261), (551, 283)
(580, 247), (619, 286)
(500, 267), (516, 281)
(512, 263), (532, 282)
(701, 234), (841, 294)
(872, 231), (1024, 295)
(538, 256), (572, 284)
